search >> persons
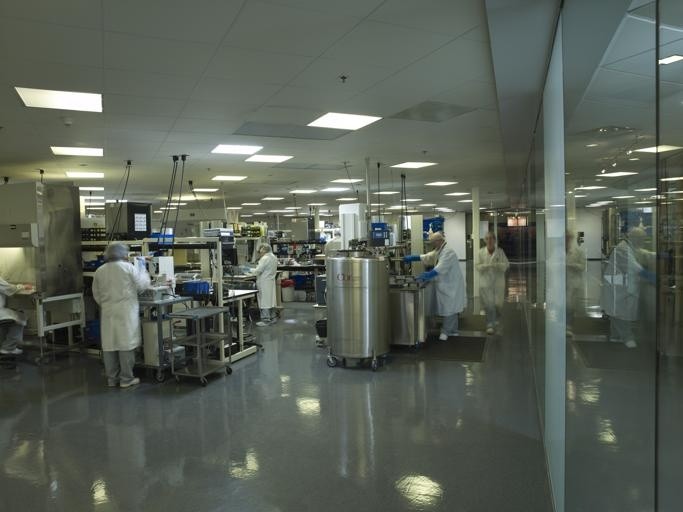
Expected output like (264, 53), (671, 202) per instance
(249, 242), (278, 327)
(403, 232), (468, 342)
(564, 230), (586, 338)
(324, 228), (341, 252)
(473, 231), (510, 335)
(599, 227), (672, 348)
(0, 270), (33, 356)
(89, 242), (151, 389)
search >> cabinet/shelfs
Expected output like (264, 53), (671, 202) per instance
(166, 306), (232, 385)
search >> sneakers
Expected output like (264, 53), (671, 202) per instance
(256, 317), (277, 327)
(439, 330), (461, 341)
(486, 320), (500, 334)
(0, 346), (23, 355)
(108, 376), (140, 388)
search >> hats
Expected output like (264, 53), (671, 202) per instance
(483, 231), (497, 242)
(256, 243), (272, 253)
(104, 243), (129, 259)
(428, 232), (446, 243)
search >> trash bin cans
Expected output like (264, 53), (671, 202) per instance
(281, 279), (295, 302)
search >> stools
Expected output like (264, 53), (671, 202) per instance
(0, 319), (16, 370)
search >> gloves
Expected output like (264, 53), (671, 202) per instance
(414, 270), (437, 281)
(403, 255), (421, 263)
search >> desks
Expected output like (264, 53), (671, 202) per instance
(221, 289), (257, 364)
